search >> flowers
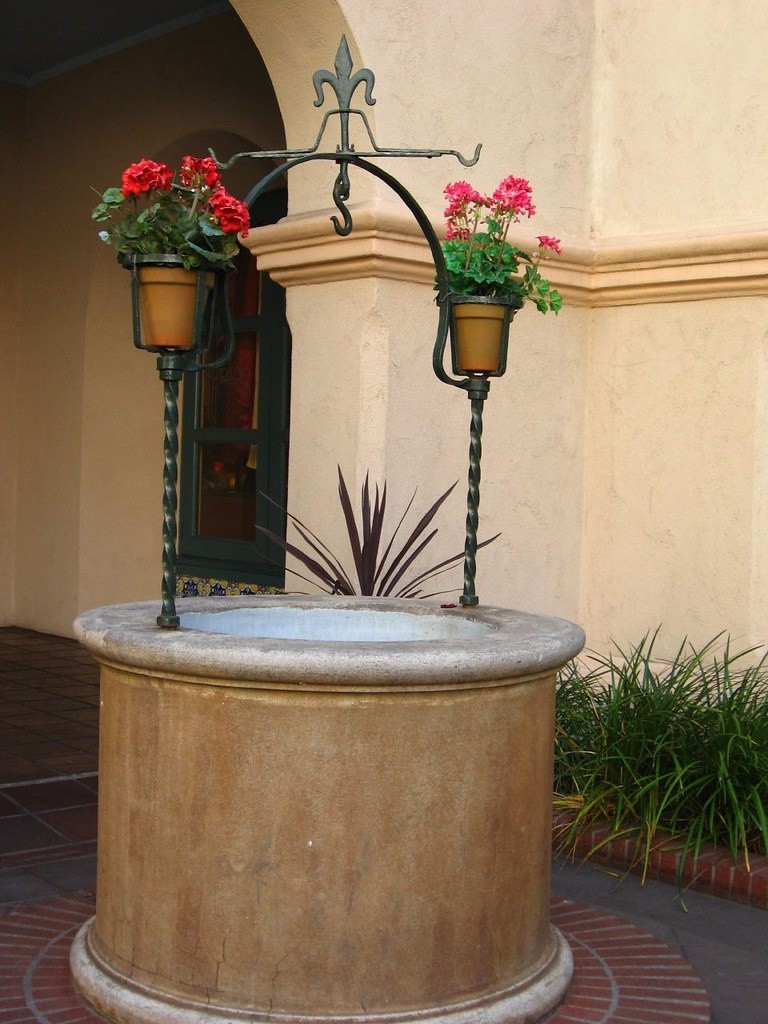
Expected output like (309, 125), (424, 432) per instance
(433, 173), (574, 316)
(88, 155), (252, 266)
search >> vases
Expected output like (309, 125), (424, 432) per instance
(132, 256), (206, 348)
(449, 291), (526, 377)
